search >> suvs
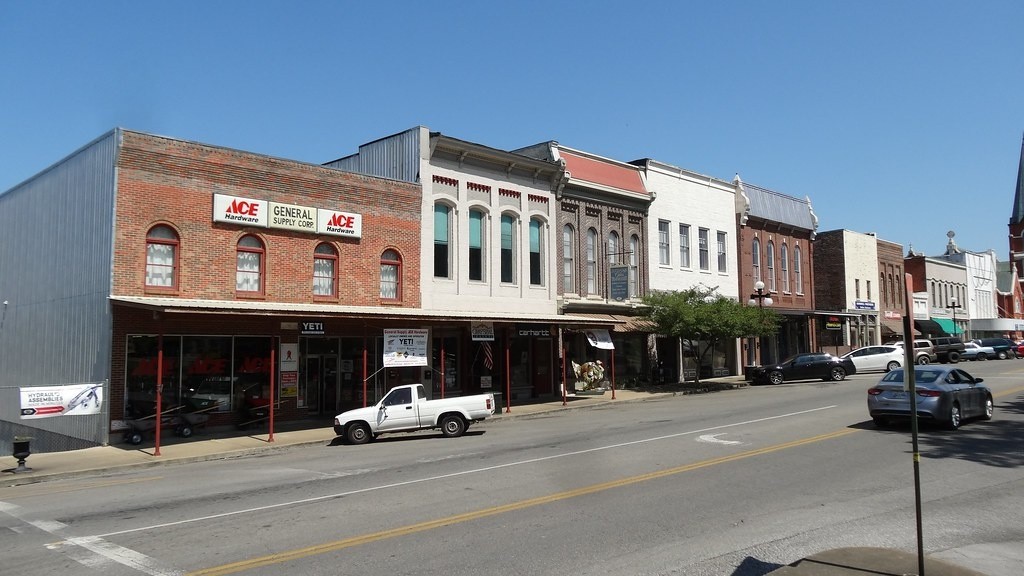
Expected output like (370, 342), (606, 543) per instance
(930, 337), (966, 364)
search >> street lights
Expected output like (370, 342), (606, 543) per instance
(951, 295), (957, 336)
(747, 280), (773, 366)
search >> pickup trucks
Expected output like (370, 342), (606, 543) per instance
(189, 376), (239, 414)
(334, 383), (495, 445)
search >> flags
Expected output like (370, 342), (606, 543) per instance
(480, 341), (493, 370)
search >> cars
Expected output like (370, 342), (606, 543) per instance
(884, 339), (937, 365)
(750, 352), (857, 386)
(963, 343), (995, 361)
(973, 338), (1014, 360)
(1015, 341), (1024, 359)
(867, 364), (993, 431)
(841, 345), (905, 372)
(1006, 339), (1018, 351)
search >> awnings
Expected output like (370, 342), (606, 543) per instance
(108, 295), (628, 326)
(931, 319), (964, 334)
(611, 315), (662, 332)
(884, 321), (921, 335)
(563, 313), (627, 333)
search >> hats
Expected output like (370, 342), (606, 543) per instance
(596, 360), (602, 364)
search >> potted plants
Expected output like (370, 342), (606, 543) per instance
(13, 435), (32, 473)
